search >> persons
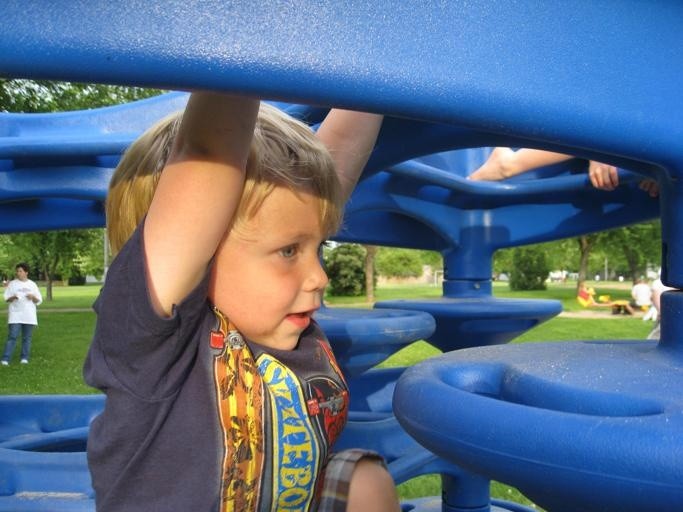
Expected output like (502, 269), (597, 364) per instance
(0, 262), (43, 366)
(627, 277), (653, 307)
(465, 143), (660, 198)
(83, 88), (407, 511)
(649, 270), (677, 331)
(577, 281), (591, 301)
